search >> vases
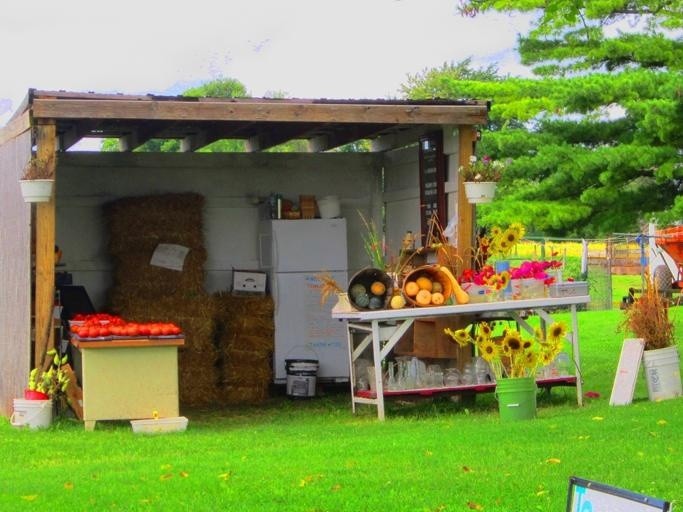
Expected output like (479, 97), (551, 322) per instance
(462, 182), (497, 204)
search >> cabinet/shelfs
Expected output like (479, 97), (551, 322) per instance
(331, 294), (590, 422)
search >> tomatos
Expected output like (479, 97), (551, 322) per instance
(69, 312), (181, 338)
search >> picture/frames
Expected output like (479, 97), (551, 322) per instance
(565, 476), (670, 512)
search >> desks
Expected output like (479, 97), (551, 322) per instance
(69, 337), (185, 432)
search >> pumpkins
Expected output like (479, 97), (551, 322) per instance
(353, 284), (381, 309)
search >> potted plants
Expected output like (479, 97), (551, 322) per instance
(18, 147), (55, 203)
(23, 348), (74, 401)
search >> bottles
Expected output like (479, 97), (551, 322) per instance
(404, 231), (413, 250)
(274, 194), (283, 220)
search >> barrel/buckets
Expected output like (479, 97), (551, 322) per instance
(10, 399), (53, 428)
(348, 266), (394, 311)
(494, 377), (537, 422)
(316, 195), (342, 218)
(285, 359), (320, 400)
(402, 265), (452, 306)
(642, 346), (683, 402)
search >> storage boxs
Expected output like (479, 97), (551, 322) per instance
(231, 269), (267, 298)
(129, 416), (188, 434)
(549, 281), (588, 298)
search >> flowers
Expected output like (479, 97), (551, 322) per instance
(456, 154), (513, 182)
(444, 319), (568, 378)
(455, 221), (562, 299)
